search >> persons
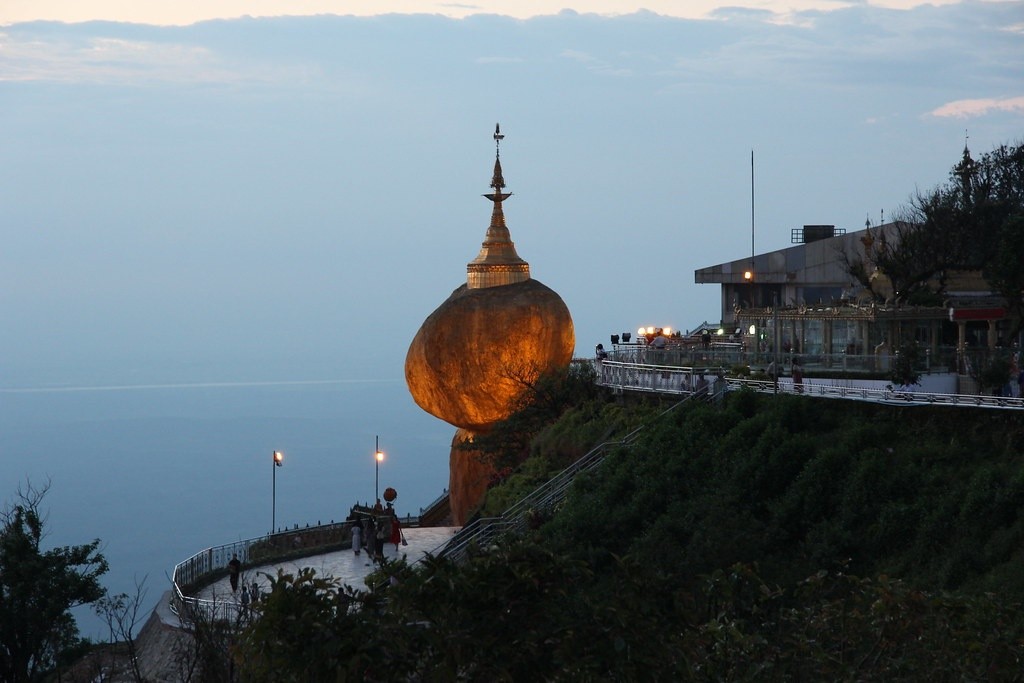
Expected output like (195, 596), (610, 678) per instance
(390, 515), (403, 552)
(846, 282), (854, 304)
(650, 332), (668, 349)
(228, 554), (240, 593)
(596, 344), (605, 360)
(351, 516), (386, 556)
